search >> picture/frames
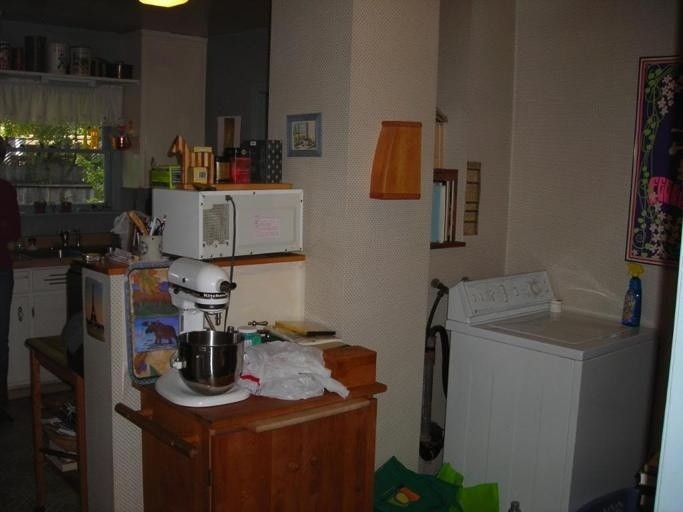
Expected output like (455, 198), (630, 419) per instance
(286, 113), (321, 157)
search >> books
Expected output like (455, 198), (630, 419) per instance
(265, 318), (344, 349)
(430, 178), (453, 243)
(636, 448), (660, 511)
(41, 418), (81, 475)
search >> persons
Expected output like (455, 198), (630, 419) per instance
(0, 135), (21, 424)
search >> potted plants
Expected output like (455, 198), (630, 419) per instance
(0, 112), (88, 213)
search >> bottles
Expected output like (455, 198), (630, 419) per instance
(0, 31), (92, 76)
(237, 325), (257, 351)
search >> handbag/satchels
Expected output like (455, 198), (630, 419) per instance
(375, 456), (499, 512)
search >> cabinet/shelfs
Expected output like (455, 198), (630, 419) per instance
(7, 266), (67, 390)
(114, 393), (387, 511)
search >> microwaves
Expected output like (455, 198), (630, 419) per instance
(151, 188), (304, 260)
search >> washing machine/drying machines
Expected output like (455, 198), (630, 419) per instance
(441, 270), (660, 512)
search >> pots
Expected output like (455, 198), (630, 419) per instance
(95, 56), (136, 78)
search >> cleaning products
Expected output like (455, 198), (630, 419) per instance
(621, 262), (647, 328)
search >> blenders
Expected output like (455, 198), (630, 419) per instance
(154, 258), (251, 407)
